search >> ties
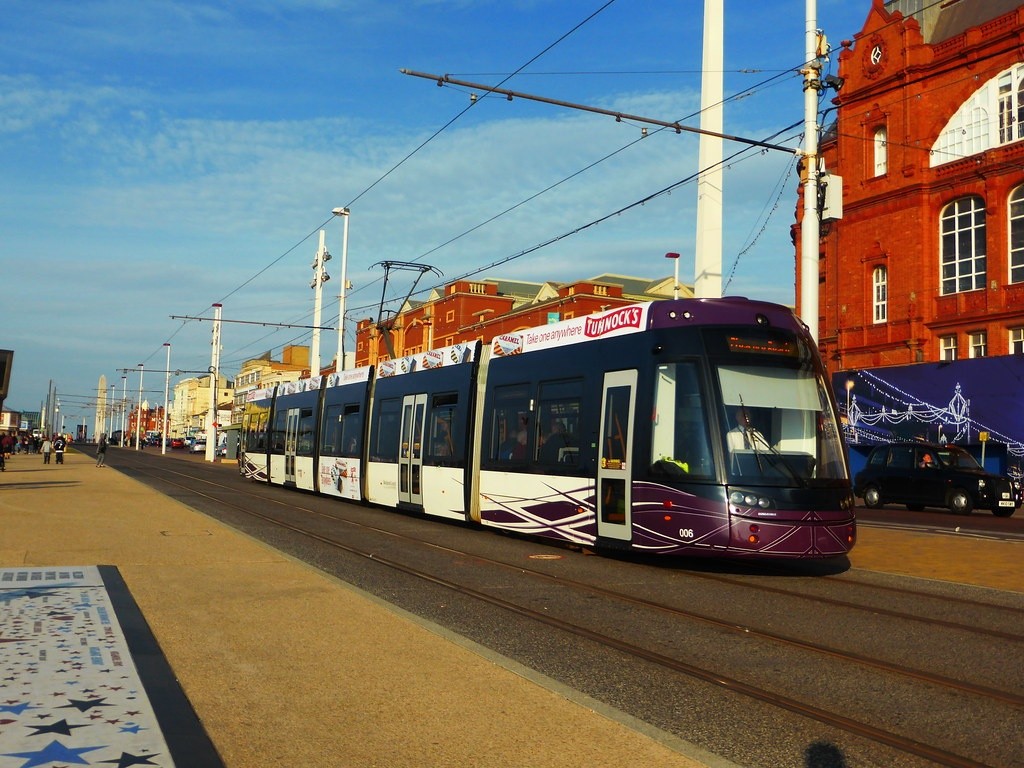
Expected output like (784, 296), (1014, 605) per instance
(743, 429), (750, 450)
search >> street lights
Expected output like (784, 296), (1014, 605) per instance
(121, 376), (127, 448)
(332, 206), (351, 372)
(109, 385), (116, 438)
(135, 364), (144, 452)
(161, 343), (172, 455)
(210, 303), (223, 462)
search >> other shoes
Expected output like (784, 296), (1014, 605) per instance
(100, 464), (106, 467)
(96, 465), (100, 467)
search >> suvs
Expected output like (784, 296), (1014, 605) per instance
(854, 441), (1023, 518)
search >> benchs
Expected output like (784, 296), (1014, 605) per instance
(298, 442), (336, 454)
(558, 447), (581, 466)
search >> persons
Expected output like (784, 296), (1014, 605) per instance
(95, 433), (108, 467)
(920, 453), (932, 468)
(141, 437), (145, 450)
(40, 433), (66, 464)
(726, 407), (769, 453)
(0, 428), (43, 472)
(502, 411), (576, 463)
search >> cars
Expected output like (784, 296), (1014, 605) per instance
(153, 438), (170, 447)
(185, 436), (196, 447)
(171, 438), (184, 449)
(191, 439), (207, 455)
(215, 443), (228, 456)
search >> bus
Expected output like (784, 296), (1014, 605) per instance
(241, 259), (859, 566)
